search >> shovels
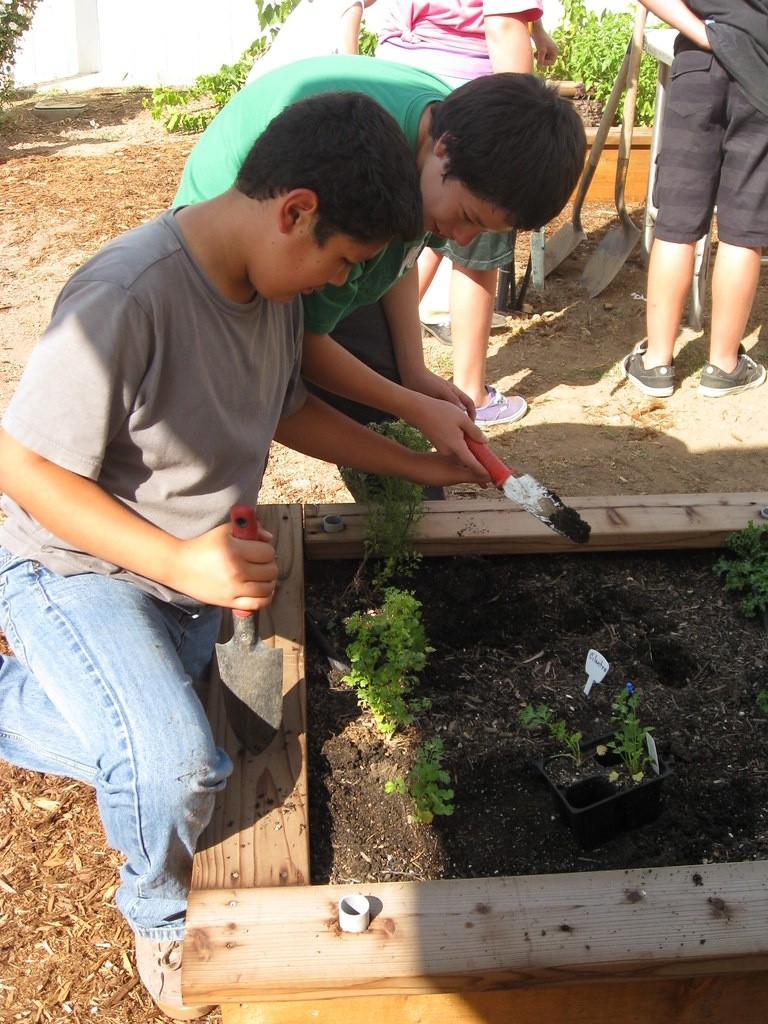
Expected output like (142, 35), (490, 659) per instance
(213, 503), (284, 754)
(462, 435), (590, 546)
(544, 33), (633, 277)
(579, 1), (644, 300)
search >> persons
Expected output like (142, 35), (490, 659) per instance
(0, 90), (493, 1024)
(170, 51), (588, 506)
(331, 0), (564, 427)
(621, 0), (768, 400)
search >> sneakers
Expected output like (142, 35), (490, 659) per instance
(135, 939), (216, 1021)
(621, 338), (674, 397)
(464, 384), (528, 425)
(697, 343), (766, 397)
